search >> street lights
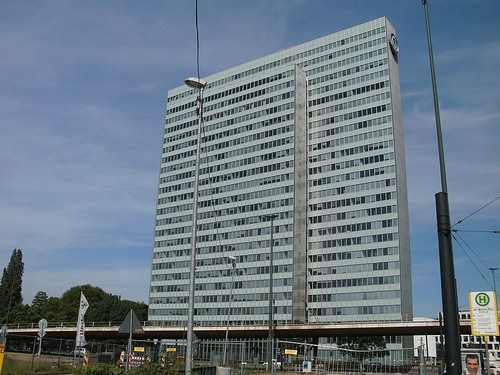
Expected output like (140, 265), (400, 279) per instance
(264, 213), (279, 370)
(488, 267), (499, 291)
(181, 76), (208, 375)
(221, 255), (236, 366)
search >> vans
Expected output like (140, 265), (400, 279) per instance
(71, 347), (89, 357)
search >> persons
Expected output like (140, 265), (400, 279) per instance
(466, 354), (479, 375)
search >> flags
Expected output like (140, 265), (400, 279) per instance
(76, 294), (89, 347)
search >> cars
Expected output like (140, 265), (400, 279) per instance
(263, 358), (282, 369)
